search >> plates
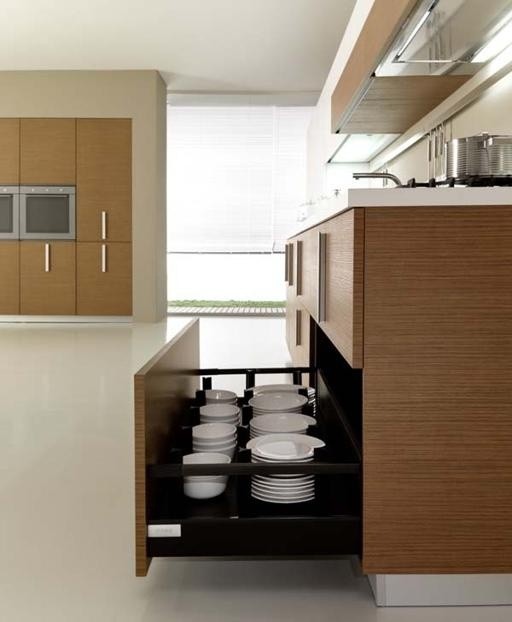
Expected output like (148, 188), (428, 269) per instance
(245, 381), (321, 502)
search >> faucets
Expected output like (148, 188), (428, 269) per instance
(352, 171), (404, 188)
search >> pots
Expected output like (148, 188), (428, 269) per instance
(443, 132), (512, 184)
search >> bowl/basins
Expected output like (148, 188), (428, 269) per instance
(182, 385), (243, 499)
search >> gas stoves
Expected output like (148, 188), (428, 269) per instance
(391, 175), (512, 189)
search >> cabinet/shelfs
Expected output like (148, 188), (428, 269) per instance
(0, 240), (76, 322)
(76, 118), (132, 242)
(330, 1), (473, 134)
(1, 118), (76, 186)
(75, 241), (133, 323)
